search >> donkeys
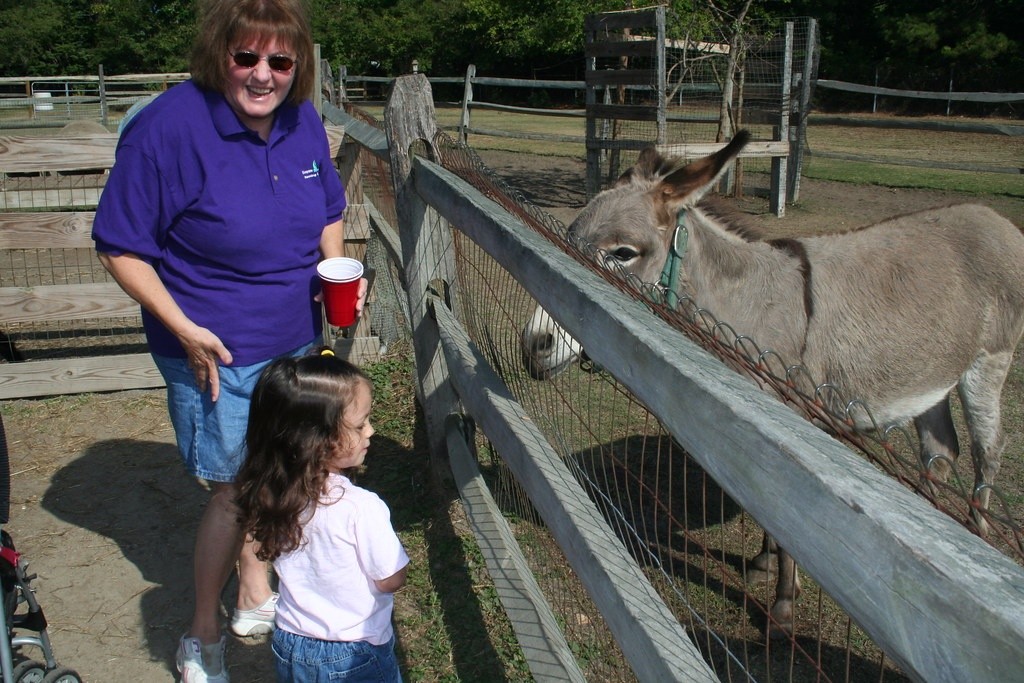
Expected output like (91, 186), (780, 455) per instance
(523, 130), (1023, 628)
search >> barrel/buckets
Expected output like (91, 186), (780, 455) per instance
(34, 93), (53, 110)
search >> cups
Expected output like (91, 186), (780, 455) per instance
(316, 258), (364, 328)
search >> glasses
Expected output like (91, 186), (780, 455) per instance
(226, 46), (299, 71)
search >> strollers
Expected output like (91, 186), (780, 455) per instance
(0, 531), (83, 683)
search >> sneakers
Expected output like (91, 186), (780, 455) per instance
(175, 630), (230, 683)
(231, 591), (279, 636)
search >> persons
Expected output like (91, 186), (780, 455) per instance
(91, 1), (368, 683)
(222, 346), (412, 683)
